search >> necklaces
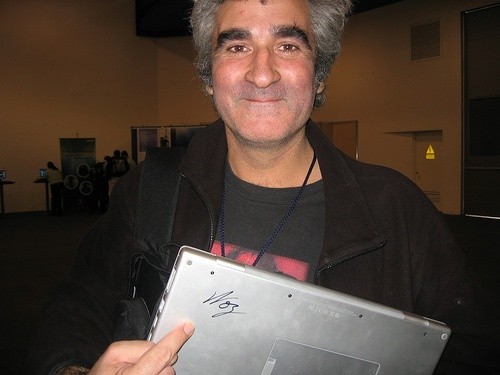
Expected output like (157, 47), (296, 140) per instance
(221, 150), (317, 268)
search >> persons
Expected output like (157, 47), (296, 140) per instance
(38, 0), (476, 375)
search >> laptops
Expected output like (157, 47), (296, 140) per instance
(146, 244), (452, 375)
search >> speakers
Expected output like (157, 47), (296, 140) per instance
(135, 0), (194, 38)
